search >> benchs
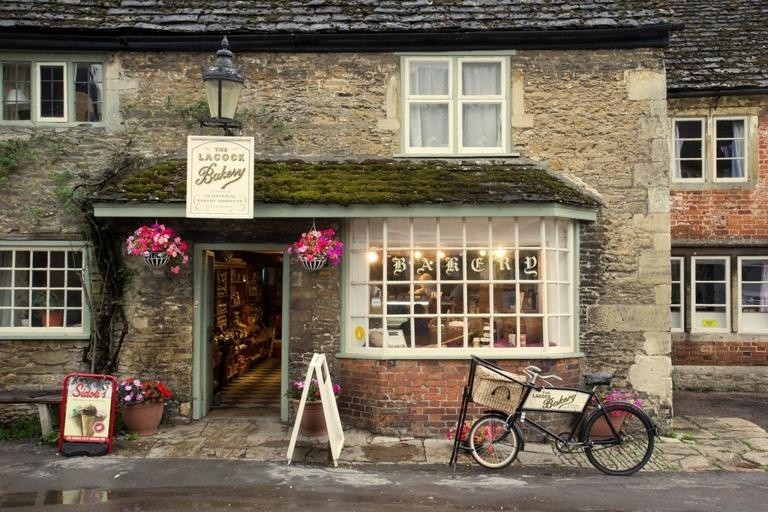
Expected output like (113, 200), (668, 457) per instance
(1, 388), (64, 438)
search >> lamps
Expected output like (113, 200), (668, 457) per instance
(4, 89), (29, 121)
(195, 33), (249, 135)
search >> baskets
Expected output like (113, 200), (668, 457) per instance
(470, 364), (527, 416)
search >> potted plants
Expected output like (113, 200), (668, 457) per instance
(30, 282), (65, 327)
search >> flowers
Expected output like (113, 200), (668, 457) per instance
(286, 225), (344, 267)
(281, 376), (341, 401)
(113, 375), (171, 412)
(589, 385), (642, 419)
(123, 222), (191, 274)
(446, 416), (496, 454)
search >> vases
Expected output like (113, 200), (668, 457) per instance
(461, 441), (480, 454)
(584, 405), (625, 439)
(297, 252), (328, 273)
(140, 250), (171, 272)
(287, 393), (339, 439)
(120, 399), (165, 437)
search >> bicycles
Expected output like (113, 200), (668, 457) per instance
(463, 361), (658, 478)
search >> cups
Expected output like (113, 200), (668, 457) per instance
(20, 318), (29, 327)
(472, 339), (480, 348)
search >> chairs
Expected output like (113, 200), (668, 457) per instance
(75, 91), (92, 122)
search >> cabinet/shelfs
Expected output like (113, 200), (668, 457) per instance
(210, 330), (272, 394)
(215, 263), (249, 335)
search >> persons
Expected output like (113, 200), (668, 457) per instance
(399, 304), (427, 337)
